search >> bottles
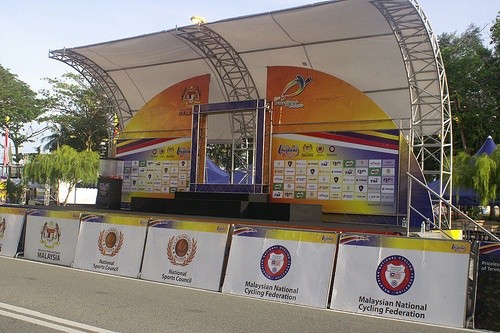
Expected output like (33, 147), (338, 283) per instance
(402, 218), (405, 227)
(405, 218), (408, 227)
(426, 218), (430, 230)
(421, 221), (426, 234)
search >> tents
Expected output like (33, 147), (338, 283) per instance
(426, 134), (500, 206)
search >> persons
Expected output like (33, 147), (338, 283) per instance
(433, 204), (448, 215)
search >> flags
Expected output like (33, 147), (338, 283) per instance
(2, 129), (10, 166)
